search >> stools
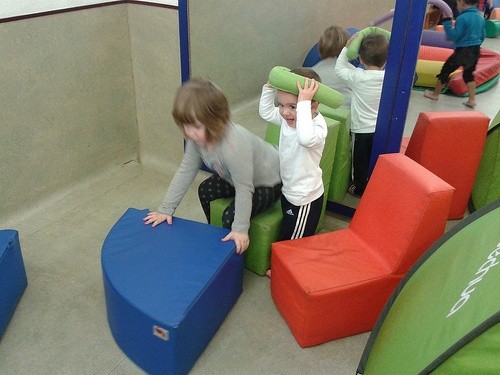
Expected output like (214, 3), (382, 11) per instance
(101, 206), (241, 375)
(0, 228), (29, 340)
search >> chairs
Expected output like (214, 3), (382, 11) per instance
(315, 103), (354, 205)
(399, 109), (491, 220)
(271, 153), (454, 350)
(209, 113), (340, 278)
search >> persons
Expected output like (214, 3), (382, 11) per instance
(142, 77), (284, 255)
(306, 25), (357, 108)
(334, 30), (388, 198)
(258, 68), (328, 277)
(424, 0), (485, 107)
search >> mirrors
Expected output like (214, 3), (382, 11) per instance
(380, 0), (500, 229)
(178, 0), (412, 218)
